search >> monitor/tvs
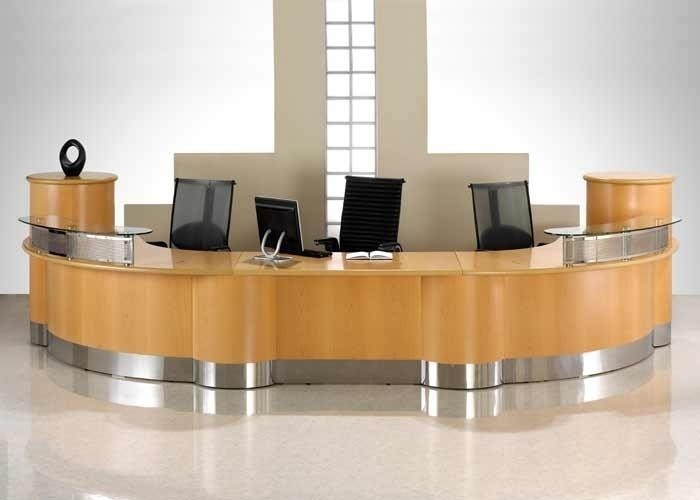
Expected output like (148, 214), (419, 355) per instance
(252, 194), (304, 263)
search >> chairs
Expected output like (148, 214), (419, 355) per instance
(315, 176), (405, 254)
(146, 178), (236, 253)
(469, 181), (547, 251)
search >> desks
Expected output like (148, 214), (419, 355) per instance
(17, 172), (683, 389)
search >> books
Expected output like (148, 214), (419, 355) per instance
(345, 250), (393, 260)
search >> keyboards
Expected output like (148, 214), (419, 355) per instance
(302, 249), (333, 260)
